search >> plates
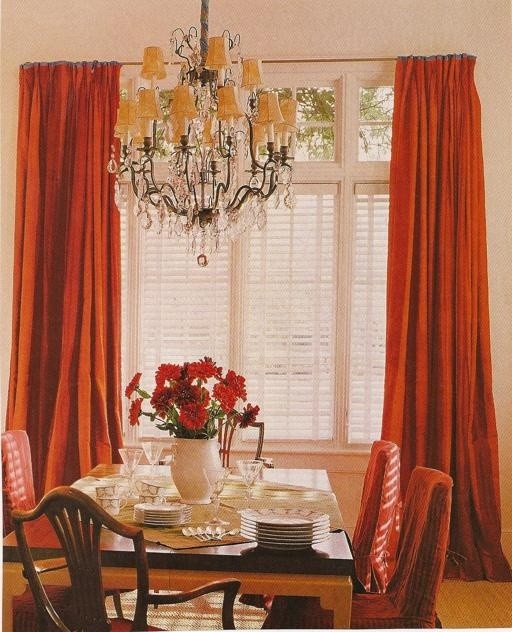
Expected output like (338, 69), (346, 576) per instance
(241, 506), (332, 550)
(133, 502), (192, 527)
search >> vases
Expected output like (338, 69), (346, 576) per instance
(169, 438), (222, 504)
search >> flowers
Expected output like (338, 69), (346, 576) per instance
(124, 356), (260, 441)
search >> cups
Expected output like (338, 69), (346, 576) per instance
(95, 485), (127, 515)
(134, 481), (168, 502)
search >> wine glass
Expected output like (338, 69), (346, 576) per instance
(203, 466), (232, 526)
(118, 448), (144, 477)
(236, 459), (263, 505)
(142, 442), (164, 469)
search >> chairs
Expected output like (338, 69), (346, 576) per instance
(210, 413), (264, 469)
(259, 440), (454, 628)
(10, 487), (241, 632)
(1, 429), (104, 632)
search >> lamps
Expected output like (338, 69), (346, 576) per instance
(107, 0), (299, 267)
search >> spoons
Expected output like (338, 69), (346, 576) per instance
(181, 527), (239, 542)
